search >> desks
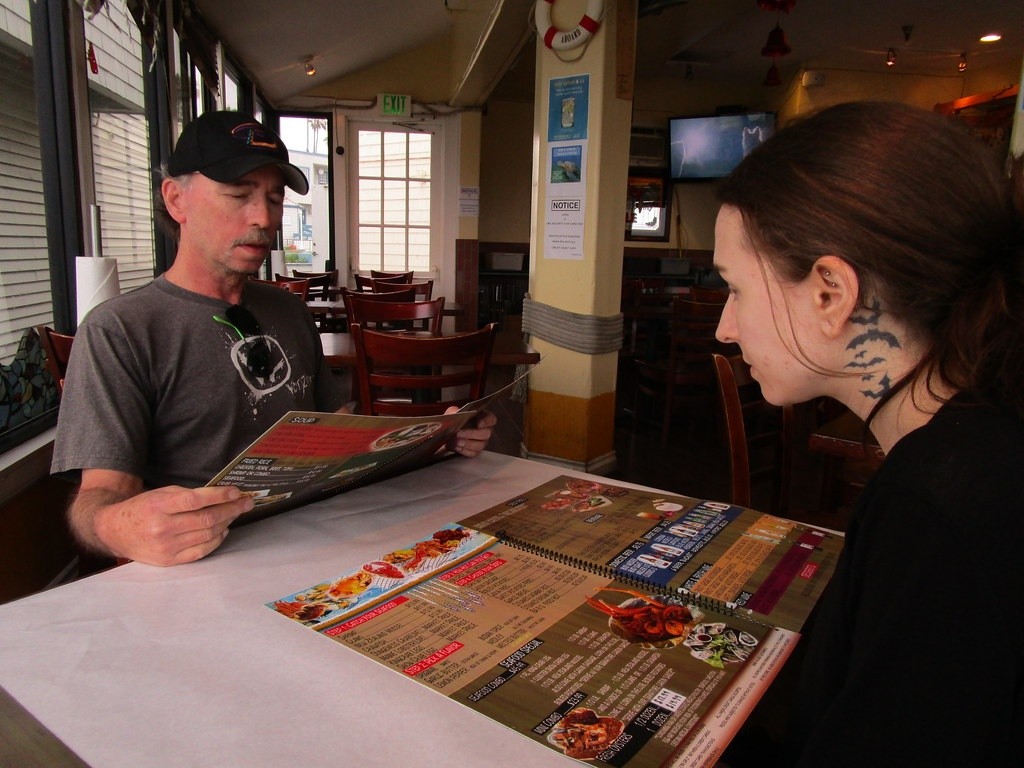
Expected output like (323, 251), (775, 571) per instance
(808, 410), (886, 460)
(320, 329), (543, 416)
(304, 299), (466, 333)
(0, 450), (848, 768)
(623, 304), (675, 436)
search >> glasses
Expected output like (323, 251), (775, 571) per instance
(226, 303), (275, 379)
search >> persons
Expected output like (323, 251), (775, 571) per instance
(48, 111), (497, 569)
(712, 97), (1020, 766)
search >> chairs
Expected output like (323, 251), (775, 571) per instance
(622, 278), (866, 531)
(43, 268), (501, 573)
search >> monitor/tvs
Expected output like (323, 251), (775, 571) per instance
(667, 111), (779, 182)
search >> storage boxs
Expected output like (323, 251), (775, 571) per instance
(485, 252), (526, 272)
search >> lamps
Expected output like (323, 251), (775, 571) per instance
(885, 47), (897, 69)
(297, 54), (317, 76)
(957, 52), (967, 73)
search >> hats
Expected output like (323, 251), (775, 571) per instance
(171, 110), (311, 198)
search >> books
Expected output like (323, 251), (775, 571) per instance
(264, 474), (848, 768)
(199, 355), (540, 528)
